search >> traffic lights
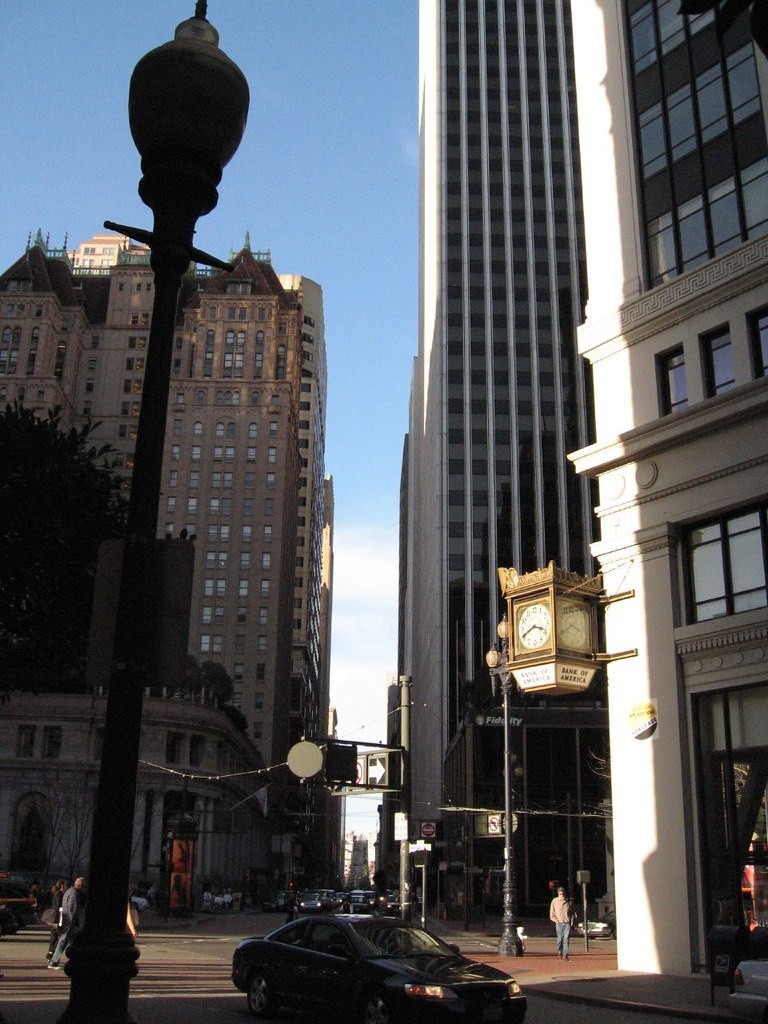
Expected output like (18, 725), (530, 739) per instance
(290, 883), (293, 887)
(549, 881), (559, 887)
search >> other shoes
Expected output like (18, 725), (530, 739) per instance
(558, 951), (569, 960)
(48, 961), (61, 970)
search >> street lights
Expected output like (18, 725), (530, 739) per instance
(62, 0), (251, 1024)
(486, 614), (522, 958)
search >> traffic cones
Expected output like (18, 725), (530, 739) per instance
(442, 909), (447, 921)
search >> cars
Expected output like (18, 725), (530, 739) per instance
(0, 874), (60, 938)
(576, 911), (615, 940)
(131, 896), (149, 912)
(312, 889), (401, 913)
(231, 912), (528, 1024)
(299, 894), (323, 911)
(263, 891), (292, 911)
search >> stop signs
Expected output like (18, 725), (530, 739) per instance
(421, 823), (436, 838)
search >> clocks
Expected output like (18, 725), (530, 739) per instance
(557, 599), (595, 651)
(513, 597), (553, 648)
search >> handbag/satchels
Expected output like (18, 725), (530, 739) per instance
(43, 909), (62, 927)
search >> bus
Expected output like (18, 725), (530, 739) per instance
(742, 841), (768, 929)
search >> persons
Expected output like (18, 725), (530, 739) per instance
(0, 850), (143, 979)
(549, 886), (579, 961)
(384, 905), (397, 916)
(284, 894), (299, 923)
(409, 890), (419, 917)
(202, 885), (254, 912)
